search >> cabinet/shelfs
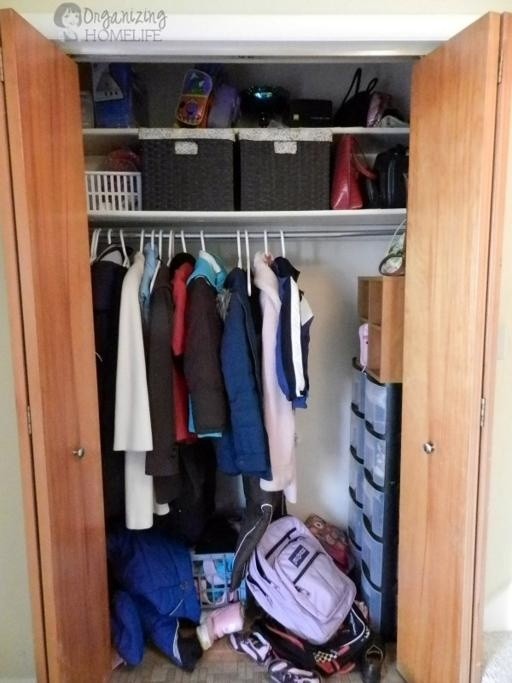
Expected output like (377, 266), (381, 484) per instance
(346, 357), (399, 647)
(57, 40), (412, 219)
(354, 272), (404, 384)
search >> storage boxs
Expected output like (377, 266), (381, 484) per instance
(235, 127), (332, 210)
(137, 125), (236, 211)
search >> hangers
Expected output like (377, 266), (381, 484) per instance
(85, 227), (299, 299)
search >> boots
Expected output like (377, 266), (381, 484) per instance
(196, 601), (245, 650)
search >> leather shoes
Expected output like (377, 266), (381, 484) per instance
(363, 634), (385, 683)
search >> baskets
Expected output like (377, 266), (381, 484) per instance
(83, 171), (142, 211)
(190, 553), (246, 608)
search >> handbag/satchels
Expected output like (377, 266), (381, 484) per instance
(330, 134), (408, 210)
(334, 92), (392, 127)
(287, 98), (333, 128)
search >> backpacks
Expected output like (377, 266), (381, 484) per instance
(245, 515), (372, 677)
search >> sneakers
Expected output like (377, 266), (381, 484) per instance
(266, 659), (323, 683)
(229, 631), (273, 665)
